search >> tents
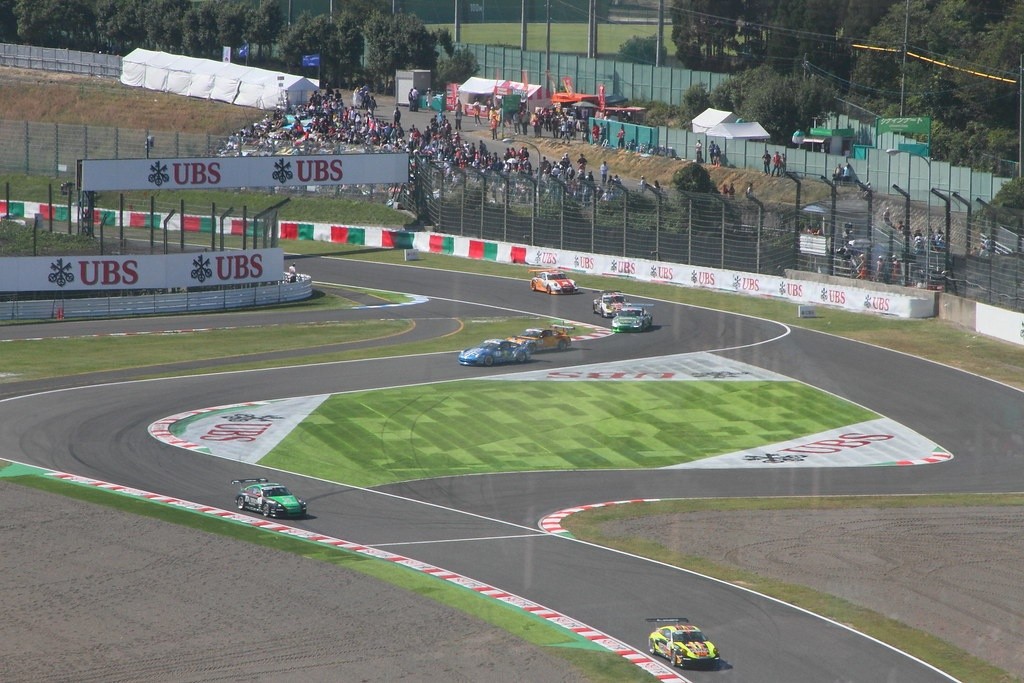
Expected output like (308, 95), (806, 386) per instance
(459, 76), (552, 123)
(606, 95), (629, 104)
(692, 108), (771, 141)
(120, 47), (320, 113)
(550, 93), (598, 103)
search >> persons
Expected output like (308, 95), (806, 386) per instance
(289, 263), (298, 282)
(723, 183), (763, 207)
(807, 207), (992, 291)
(761, 150), (786, 177)
(216, 83), (662, 205)
(696, 139), (721, 167)
(835, 163), (852, 185)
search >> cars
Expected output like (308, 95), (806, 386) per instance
(595, 290), (630, 319)
(529, 266), (578, 295)
(460, 338), (532, 367)
(645, 616), (720, 669)
(508, 323), (575, 353)
(613, 306), (654, 333)
(230, 477), (308, 519)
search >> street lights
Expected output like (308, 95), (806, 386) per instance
(501, 139), (545, 218)
(886, 150), (933, 290)
